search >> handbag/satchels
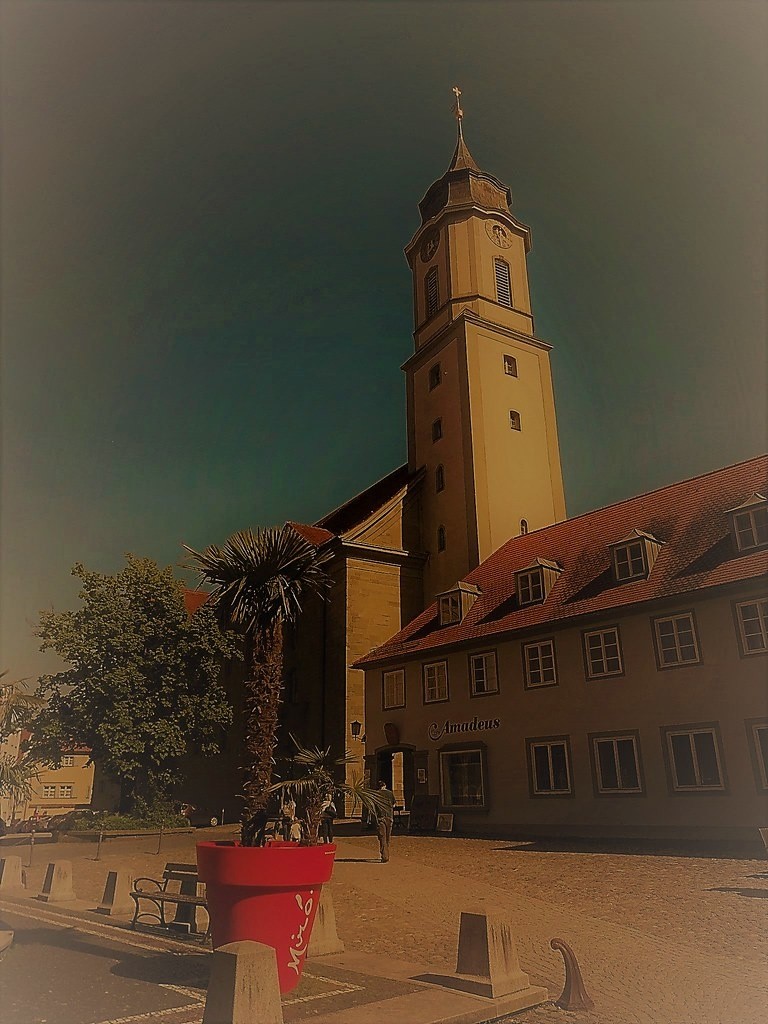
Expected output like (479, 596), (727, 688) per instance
(325, 801), (336, 818)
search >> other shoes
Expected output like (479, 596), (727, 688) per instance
(381, 858), (389, 863)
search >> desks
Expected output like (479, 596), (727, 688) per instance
(392, 806), (406, 828)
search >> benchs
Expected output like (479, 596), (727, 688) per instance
(130, 862), (211, 946)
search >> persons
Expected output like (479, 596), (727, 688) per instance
(281, 793), (336, 844)
(375, 780), (396, 863)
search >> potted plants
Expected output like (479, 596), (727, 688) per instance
(178, 526), (395, 996)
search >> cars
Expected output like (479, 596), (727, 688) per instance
(0, 809), (108, 834)
(181, 802), (221, 827)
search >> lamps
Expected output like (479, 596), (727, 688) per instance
(351, 719), (366, 743)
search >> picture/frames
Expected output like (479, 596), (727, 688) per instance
(435, 813), (454, 833)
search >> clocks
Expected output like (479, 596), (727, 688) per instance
(485, 219), (514, 249)
(419, 227), (441, 263)
